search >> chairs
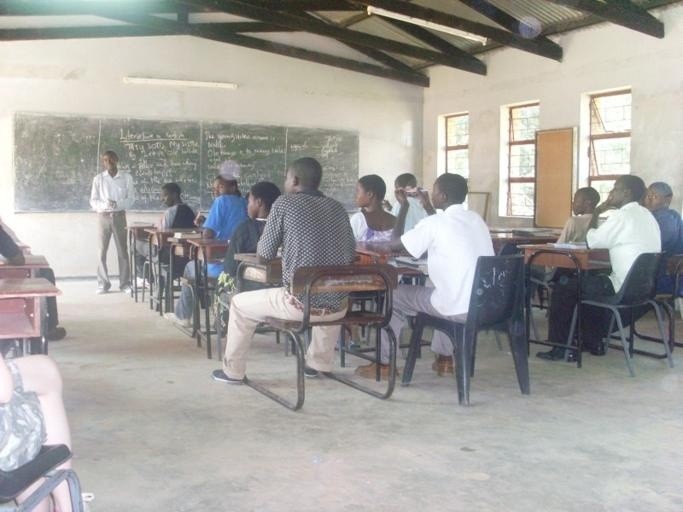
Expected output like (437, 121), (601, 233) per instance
(0, 444), (84, 512)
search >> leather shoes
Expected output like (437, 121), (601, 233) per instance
(579, 337), (605, 356)
(536, 342), (582, 362)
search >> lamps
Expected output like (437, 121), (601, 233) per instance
(122, 76), (239, 90)
(366, 5), (492, 47)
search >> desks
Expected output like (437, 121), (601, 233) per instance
(0, 254), (50, 279)
(18, 243), (31, 256)
(0, 277), (62, 357)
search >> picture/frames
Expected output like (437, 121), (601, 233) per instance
(534, 127), (577, 228)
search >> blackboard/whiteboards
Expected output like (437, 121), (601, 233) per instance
(12, 112), (361, 215)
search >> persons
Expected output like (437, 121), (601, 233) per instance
(212, 158), (356, 383)
(0, 224), (66, 341)
(536, 175), (683, 362)
(0, 350), (74, 512)
(156, 174), (279, 335)
(90, 150), (135, 293)
(349, 173), (427, 348)
(355, 174), (495, 381)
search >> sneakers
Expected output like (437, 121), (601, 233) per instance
(97, 278), (195, 328)
(305, 357), (455, 378)
(211, 369), (245, 384)
